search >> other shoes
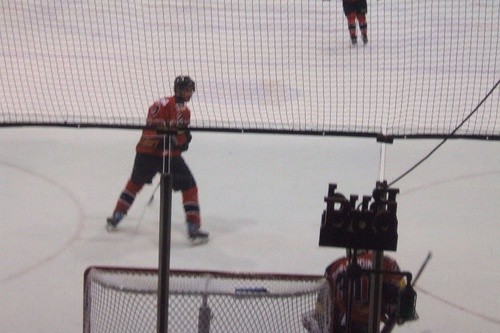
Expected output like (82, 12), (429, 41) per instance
(364, 37), (368, 44)
(351, 36), (357, 43)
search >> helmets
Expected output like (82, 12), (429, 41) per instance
(174, 76), (194, 92)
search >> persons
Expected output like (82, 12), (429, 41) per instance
(106, 75), (208, 239)
(343, 0), (368, 44)
(301, 248), (405, 333)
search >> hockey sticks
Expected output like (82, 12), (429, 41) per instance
(382, 251), (432, 333)
(145, 178), (163, 207)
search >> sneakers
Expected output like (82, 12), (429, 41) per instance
(105, 218), (117, 234)
(188, 225), (210, 245)
